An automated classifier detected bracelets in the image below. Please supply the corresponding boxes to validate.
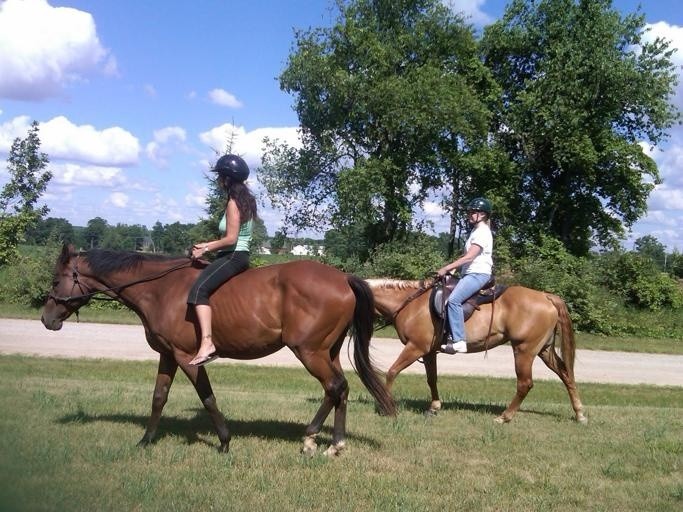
[205,246,209,253]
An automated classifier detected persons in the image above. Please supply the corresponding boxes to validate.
[436,198,494,353]
[187,154,258,369]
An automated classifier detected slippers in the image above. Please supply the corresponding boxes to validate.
[188,354,217,368]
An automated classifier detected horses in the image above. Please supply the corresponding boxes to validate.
[349,278,587,425]
[41,237,401,460]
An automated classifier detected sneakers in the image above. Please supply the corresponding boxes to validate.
[440,340,467,352]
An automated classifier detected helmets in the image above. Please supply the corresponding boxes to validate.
[210,154,249,180]
[467,198,491,212]
